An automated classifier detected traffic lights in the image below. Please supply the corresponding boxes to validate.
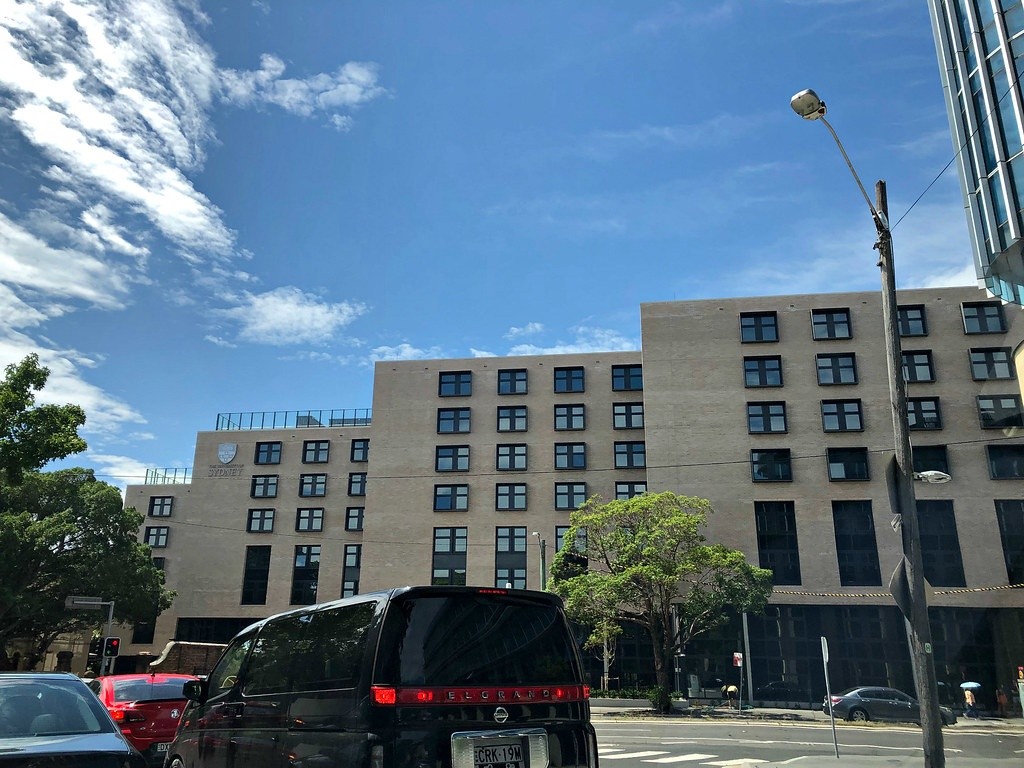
[103,637,120,658]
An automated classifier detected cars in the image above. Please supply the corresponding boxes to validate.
[0,671,151,768]
[87,671,207,761]
[823,684,959,727]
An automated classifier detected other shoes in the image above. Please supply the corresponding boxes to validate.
[963,713,968,719]
[975,718,980,721]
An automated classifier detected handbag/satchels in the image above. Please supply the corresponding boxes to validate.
[999,695,1005,704]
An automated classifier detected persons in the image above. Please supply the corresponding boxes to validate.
[963,688,978,720]
[720,685,728,699]
[726,685,738,709]
[1010,679,1021,718]
[996,683,1007,717]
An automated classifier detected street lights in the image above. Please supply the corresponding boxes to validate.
[790,87,948,768]
[533,531,546,593]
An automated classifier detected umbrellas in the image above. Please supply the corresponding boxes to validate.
[959,682,981,688]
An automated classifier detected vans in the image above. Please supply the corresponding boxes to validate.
[160,582,599,768]
